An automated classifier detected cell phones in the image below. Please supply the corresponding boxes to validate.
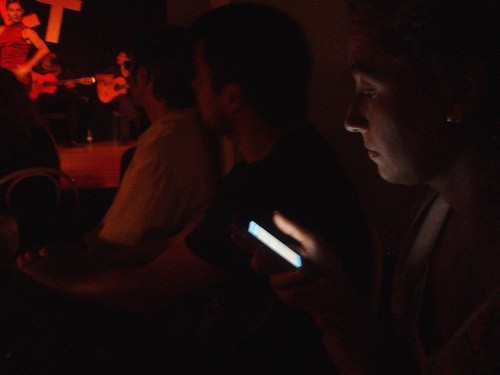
[245,220,312,273]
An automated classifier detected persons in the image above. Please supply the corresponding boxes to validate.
[230,0,500,375]
[0,0,372,375]
